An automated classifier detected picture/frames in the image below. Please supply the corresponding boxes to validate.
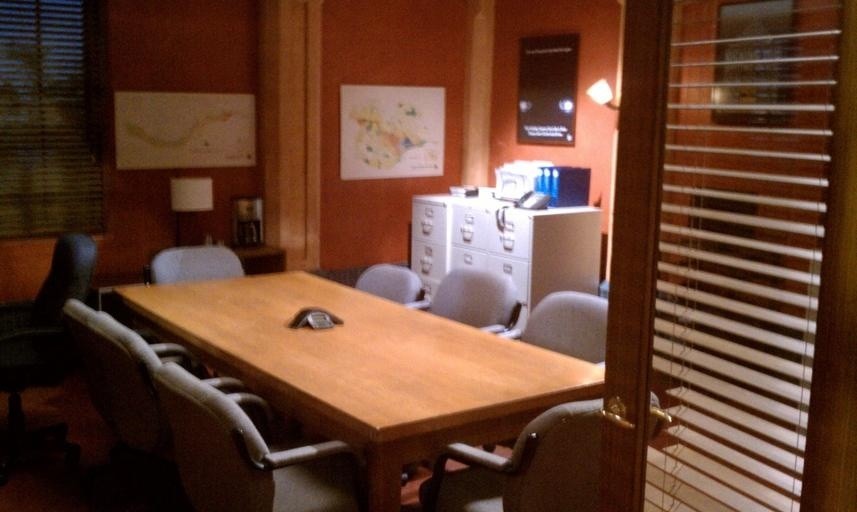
[515,32,579,145]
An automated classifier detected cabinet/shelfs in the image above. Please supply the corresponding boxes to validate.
[409,186,603,320]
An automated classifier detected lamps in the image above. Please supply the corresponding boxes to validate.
[168,176,213,247]
[585,78,617,110]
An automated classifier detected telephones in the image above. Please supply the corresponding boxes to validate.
[514,190,548,209]
[290,306,343,330]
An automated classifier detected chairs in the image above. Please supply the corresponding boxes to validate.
[71,246,607,511]
